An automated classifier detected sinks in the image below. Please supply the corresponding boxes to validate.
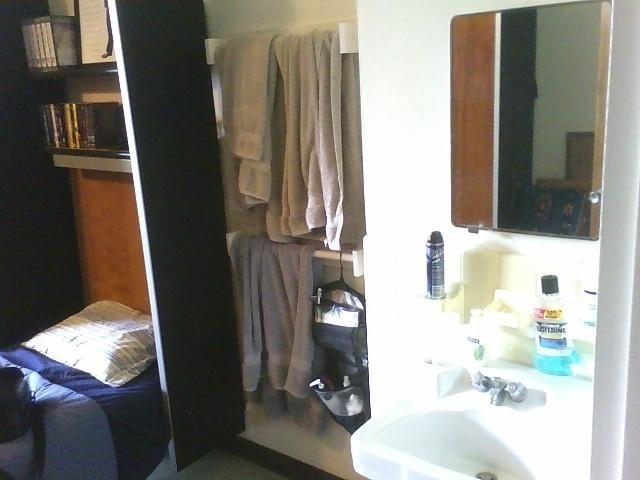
[350,360,594,480]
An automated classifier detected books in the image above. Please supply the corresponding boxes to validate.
[20,14,80,72]
[36,100,119,150]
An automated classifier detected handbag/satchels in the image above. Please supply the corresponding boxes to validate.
[0,367,33,443]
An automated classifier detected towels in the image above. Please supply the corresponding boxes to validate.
[224,233,319,403]
[217,25,279,215]
[272,28,350,252]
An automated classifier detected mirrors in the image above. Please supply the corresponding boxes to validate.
[448,0,615,243]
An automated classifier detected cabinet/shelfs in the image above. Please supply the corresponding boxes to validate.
[26,61,135,174]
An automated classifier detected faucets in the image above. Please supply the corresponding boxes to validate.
[470,372,527,407]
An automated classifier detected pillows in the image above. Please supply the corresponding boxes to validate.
[19,299,163,394]
